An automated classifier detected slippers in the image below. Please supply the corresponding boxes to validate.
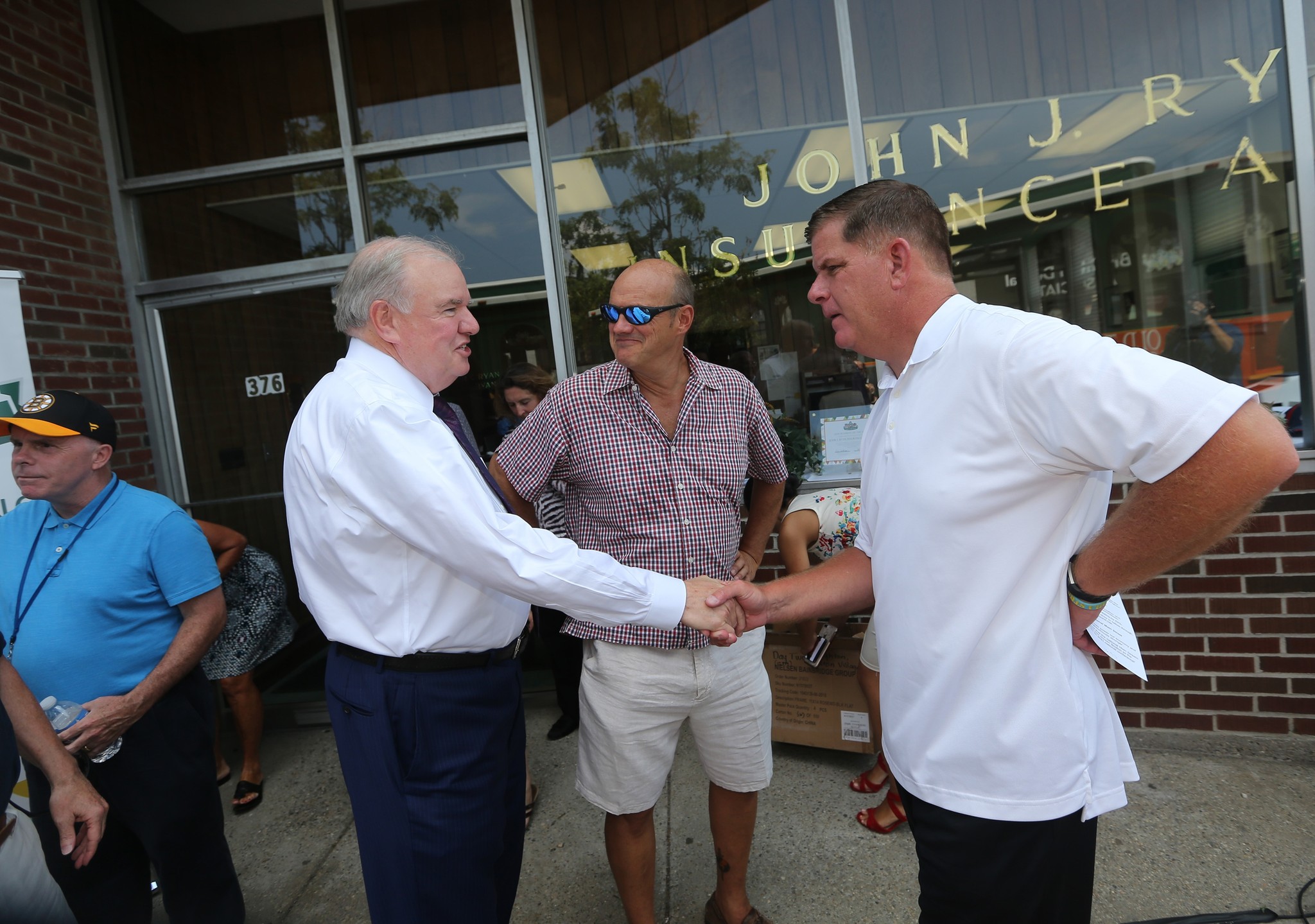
[217,771,231,787]
[231,779,264,812]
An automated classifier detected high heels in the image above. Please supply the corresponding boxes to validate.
[850,751,895,793]
[856,790,908,834]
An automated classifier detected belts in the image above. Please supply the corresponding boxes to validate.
[328,620,532,672]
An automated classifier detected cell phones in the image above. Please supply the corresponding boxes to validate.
[802,623,837,667]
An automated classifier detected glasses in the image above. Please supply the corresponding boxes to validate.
[599,302,682,326]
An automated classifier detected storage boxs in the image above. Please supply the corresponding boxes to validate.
[763,622,876,754]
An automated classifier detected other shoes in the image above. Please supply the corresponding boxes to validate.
[547,709,580,740]
[703,890,774,924]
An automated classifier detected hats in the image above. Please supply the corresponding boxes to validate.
[0,389,119,450]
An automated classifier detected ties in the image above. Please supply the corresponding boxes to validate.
[431,394,516,516]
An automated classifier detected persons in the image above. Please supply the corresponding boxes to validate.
[0,392,247,924]
[744,472,913,832]
[283,232,748,924]
[701,179,1300,924]
[491,256,788,924]
[1045,300,1064,320]
[0,632,110,924]
[497,361,583,743]
[186,517,286,811]
[1163,286,1246,390]
[728,321,870,433]
[522,749,533,830]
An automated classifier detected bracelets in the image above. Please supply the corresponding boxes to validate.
[1060,552,1120,612]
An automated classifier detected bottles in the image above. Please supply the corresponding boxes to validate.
[39,696,122,763]
